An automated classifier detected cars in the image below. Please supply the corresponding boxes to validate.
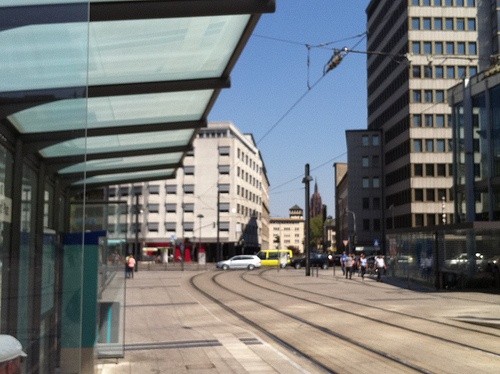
[216,254,261,270]
[291,253,342,271]
[364,251,496,281]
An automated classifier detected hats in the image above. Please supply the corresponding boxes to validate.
[0,334,28,363]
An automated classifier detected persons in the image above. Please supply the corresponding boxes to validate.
[0,333,28,374]
[421,256,433,281]
[125,255,135,279]
[374,254,386,282]
[110,252,121,265]
[340,251,366,280]
[390,254,410,277]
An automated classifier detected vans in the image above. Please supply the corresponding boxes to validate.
[257,250,293,266]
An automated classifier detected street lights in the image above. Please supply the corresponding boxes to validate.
[134,191,142,271]
[343,208,357,244]
[197,214,205,247]
